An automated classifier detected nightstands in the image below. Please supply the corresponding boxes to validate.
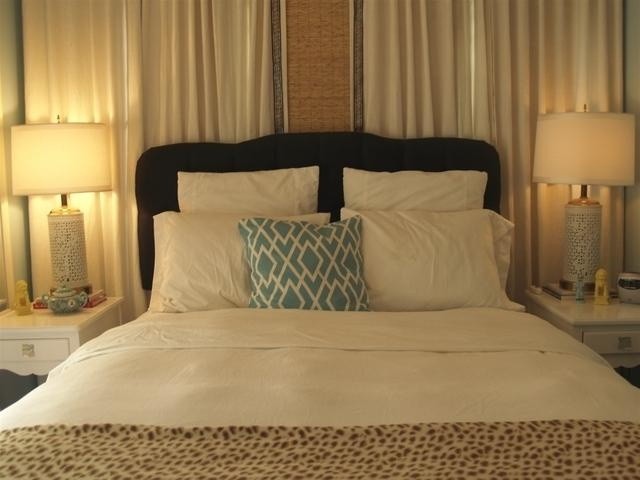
[525,286,640,368]
[0,296,125,376]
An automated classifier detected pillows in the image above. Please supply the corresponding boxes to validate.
[343,166,488,211]
[340,208,526,312]
[147,210,330,313]
[177,166,319,214]
[239,215,371,312]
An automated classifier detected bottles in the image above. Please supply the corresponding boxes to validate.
[576,273,585,303]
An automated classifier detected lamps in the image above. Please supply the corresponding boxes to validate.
[532,104,636,301]
[11,114,112,307]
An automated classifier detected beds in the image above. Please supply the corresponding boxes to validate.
[0,131,640,479]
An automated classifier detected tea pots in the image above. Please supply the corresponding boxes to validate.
[42,284,88,314]
